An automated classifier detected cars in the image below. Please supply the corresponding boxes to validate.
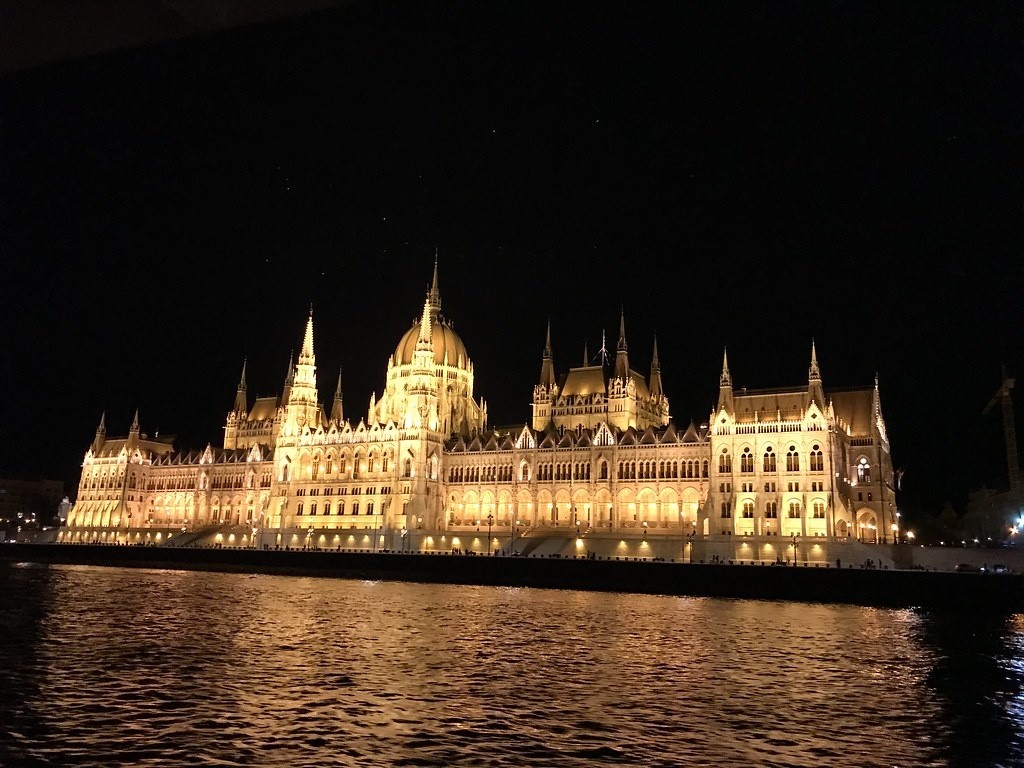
[954,562,976,572]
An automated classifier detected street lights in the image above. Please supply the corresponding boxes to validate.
[307,517,314,552]
[372,511,377,553]
[686,530,696,564]
[487,509,494,557]
[790,534,799,567]
[251,518,259,550]
